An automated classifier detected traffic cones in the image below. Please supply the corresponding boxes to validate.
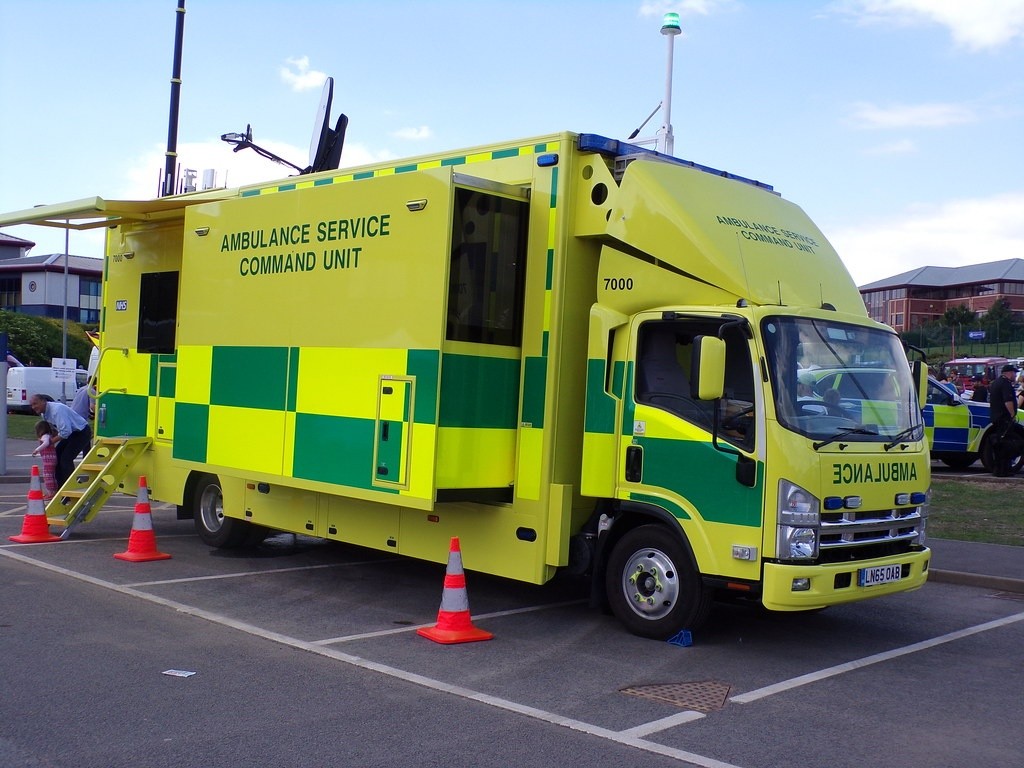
[8,465,76,544]
[416,537,494,644]
[112,476,176,562]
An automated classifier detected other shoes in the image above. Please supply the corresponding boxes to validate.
[993,470,1014,477]
[43,493,55,501]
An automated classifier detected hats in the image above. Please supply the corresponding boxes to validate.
[968,373,982,382]
[1001,365,1020,372]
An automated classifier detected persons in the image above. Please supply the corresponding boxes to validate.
[796,375,855,418]
[31,375,97,502]
[925,364,1023,478]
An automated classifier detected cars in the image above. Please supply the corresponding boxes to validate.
[944,355,1024,402]
[796,365,1024,477]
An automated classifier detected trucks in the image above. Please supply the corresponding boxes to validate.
[5,366,88,416]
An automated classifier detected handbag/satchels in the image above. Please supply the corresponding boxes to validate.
[955,379,966,394]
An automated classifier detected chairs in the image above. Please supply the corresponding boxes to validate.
[641,332,712,420]
[726,326,756,402]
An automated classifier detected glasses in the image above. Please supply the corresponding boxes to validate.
[950,372,953,374]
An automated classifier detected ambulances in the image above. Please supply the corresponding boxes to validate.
[0,11,933,640]
